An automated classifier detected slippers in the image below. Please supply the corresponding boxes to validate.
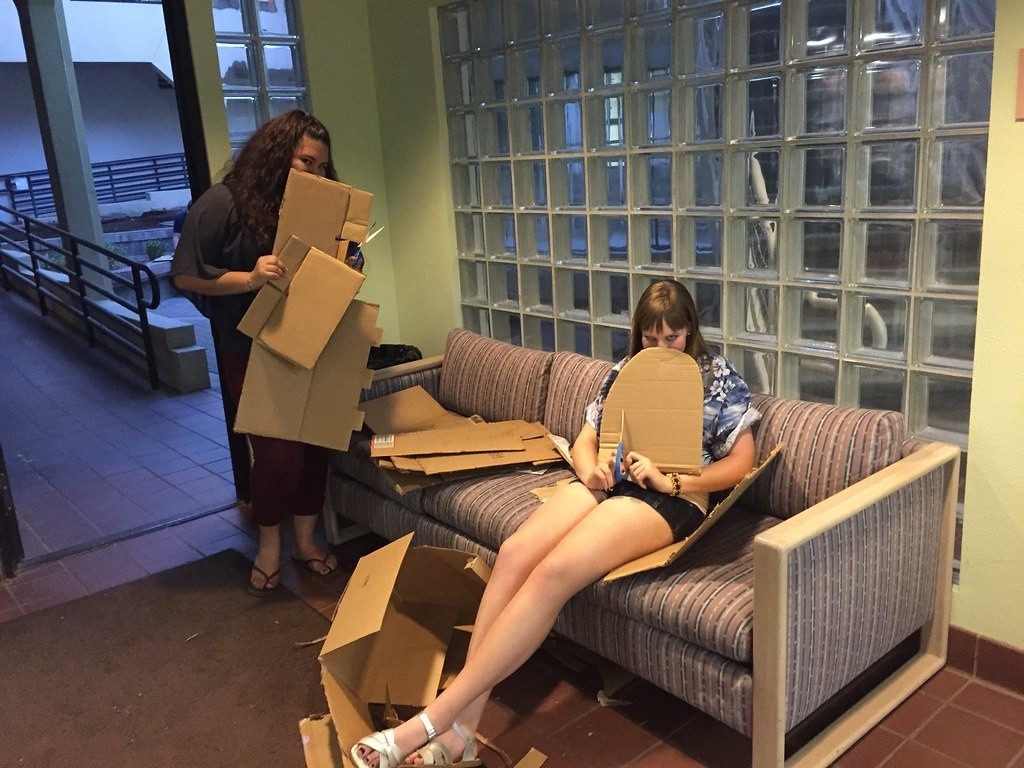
[290,550,340,575]
[247,560,281,595]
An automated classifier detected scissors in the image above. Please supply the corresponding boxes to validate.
[614,409,626,482]
[346,221,386,268]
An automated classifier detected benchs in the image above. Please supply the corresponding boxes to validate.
[325,327,961,768]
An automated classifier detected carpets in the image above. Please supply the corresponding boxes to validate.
[0,548,336,768]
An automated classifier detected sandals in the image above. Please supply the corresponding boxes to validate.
[351,712,437,768]
[398,722,482,768]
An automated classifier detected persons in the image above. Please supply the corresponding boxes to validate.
[167,109,364,598]
[349,279,763,768]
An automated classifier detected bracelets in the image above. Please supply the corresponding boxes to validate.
[666,472,683,496]
[247,271,253,290]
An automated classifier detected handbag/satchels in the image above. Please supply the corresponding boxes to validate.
[366,344,422,370]
